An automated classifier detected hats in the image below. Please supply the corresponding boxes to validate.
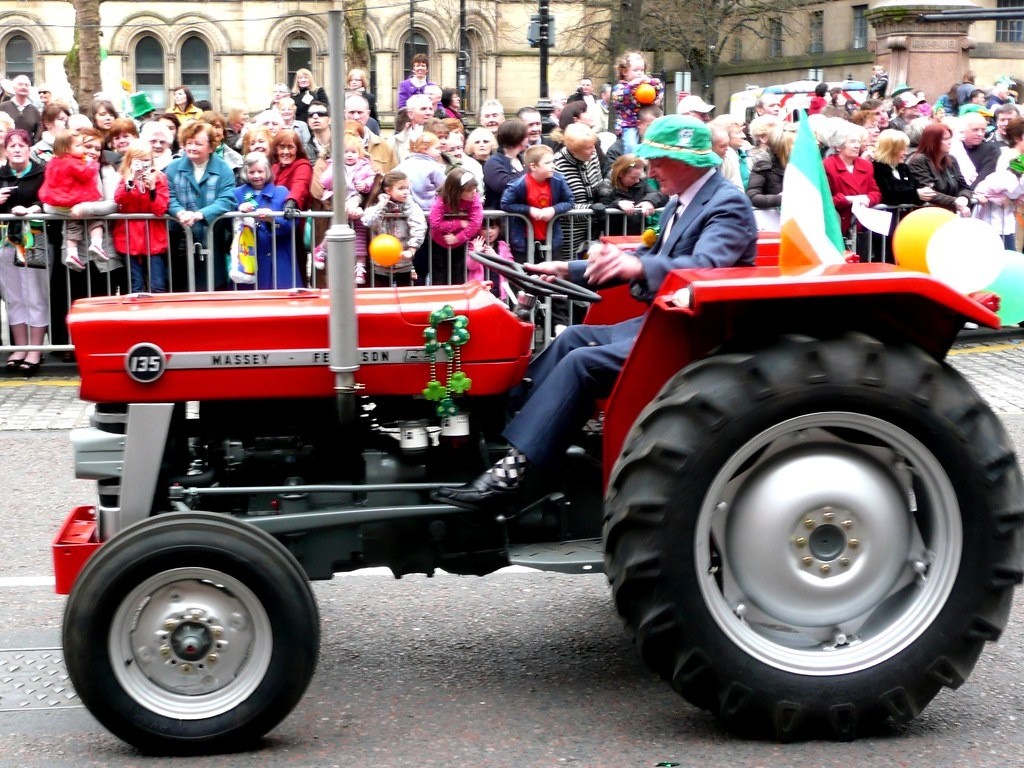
[128,91,158,118]
[634,114,724,166]
[891,83,914,98]
[676,95,718,116]
[893,91,927,111]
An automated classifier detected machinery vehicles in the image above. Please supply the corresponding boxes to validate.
[49,10,1024,762]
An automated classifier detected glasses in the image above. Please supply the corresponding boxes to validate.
[37,90,52,95]
[306,110,330,119]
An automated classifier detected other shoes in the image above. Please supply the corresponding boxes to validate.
[64,255,85,270]
[314,245,327,270]
[88,244,109,264]
[964,321,979,330]
[355,265,366,284]
[5,352,45,375]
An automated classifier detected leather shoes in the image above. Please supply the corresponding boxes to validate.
[432,472,530,515]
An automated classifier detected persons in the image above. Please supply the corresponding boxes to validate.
[431,115,755,512]
[0,0,1024,376]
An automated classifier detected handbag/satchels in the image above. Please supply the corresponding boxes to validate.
[228,217,259,284]
[14,231,50,269]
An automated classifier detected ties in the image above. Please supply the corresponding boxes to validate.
[671,202,683,230]
